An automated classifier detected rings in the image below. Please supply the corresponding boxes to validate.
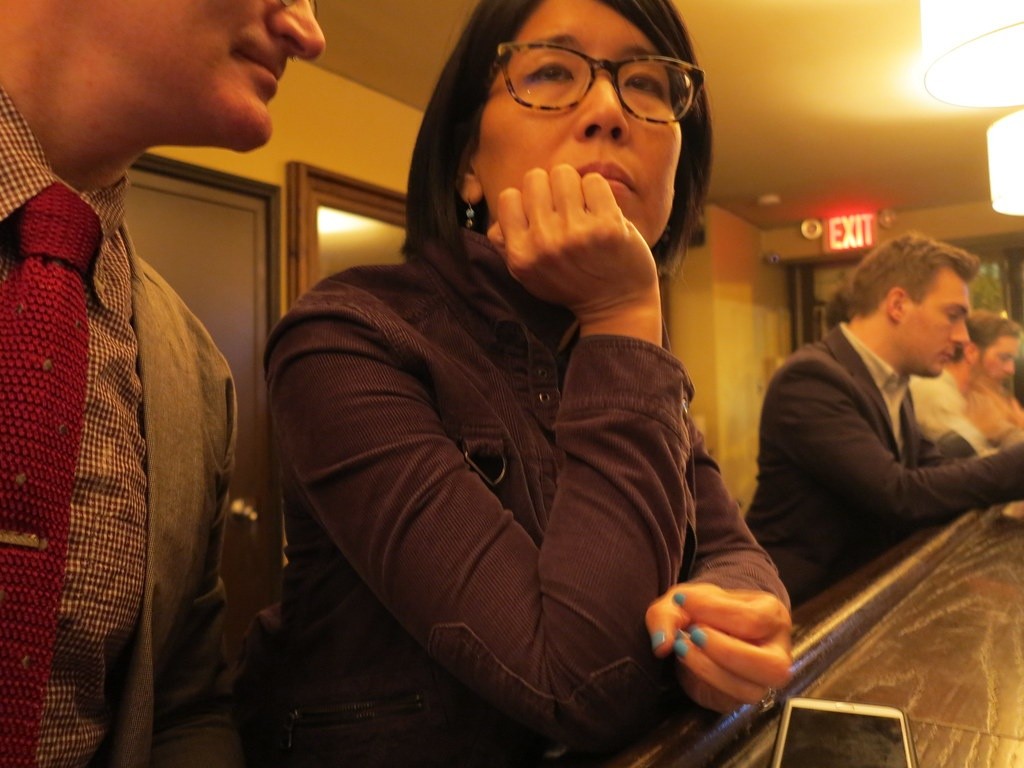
[756,687,774,713]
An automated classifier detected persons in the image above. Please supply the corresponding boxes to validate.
[0,1,327,768]
[743,228,1024,606]
[260,0,799,768]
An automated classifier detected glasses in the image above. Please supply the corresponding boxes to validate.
[485,42,706,124]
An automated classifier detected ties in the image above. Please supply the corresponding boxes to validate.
[0,183,103,768]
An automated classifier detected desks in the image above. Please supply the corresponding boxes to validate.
[609,495,1024,768]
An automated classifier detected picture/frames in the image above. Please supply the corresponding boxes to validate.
[285,160,409,314]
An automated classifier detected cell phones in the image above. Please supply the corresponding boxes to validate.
[770,696,920,768]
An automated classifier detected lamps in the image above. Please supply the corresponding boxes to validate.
[917,0,1024,217]
[798,209,897,252]
